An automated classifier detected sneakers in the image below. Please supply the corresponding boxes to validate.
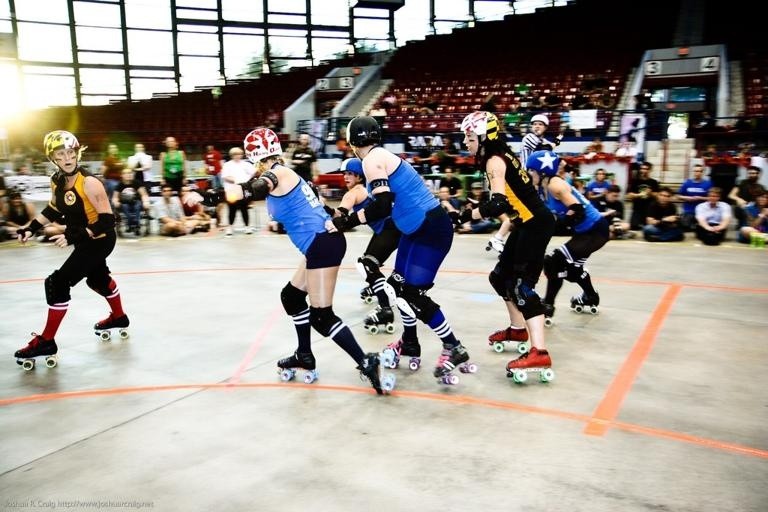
[226,227,233,235]
[243,225,252,234]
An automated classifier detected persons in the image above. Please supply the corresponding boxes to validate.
[306,158,396,324]
[269,82,767,245]
[14,129,131,358]
[525,148,610,318]
[325,115,477,377]
[227,125,384,394]
[1,137,289,244]
[441,110,558,372]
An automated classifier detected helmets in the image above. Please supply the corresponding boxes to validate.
[339,158,365,178]
[526,150,560,176]
[530,114,550,127]
[346,116,382,147]
[43,130,81,162]
[460,110,501,142]
[244,128,283,164]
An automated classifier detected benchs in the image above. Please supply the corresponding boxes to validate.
[366,65,632,134]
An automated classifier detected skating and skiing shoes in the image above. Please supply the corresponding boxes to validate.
[382,331,421,371]
[434,340,477,385]
[540,299,554,327]
[363,306,395,335]
[570,293,599,315]
[360,286,377,304]
[94,312,129,341]
[277,349,319,384]
[15,332,57,371]
[506,347,554,383]
[485,235,506,260]
[356,349,395,395]
[488,326,529,354]
